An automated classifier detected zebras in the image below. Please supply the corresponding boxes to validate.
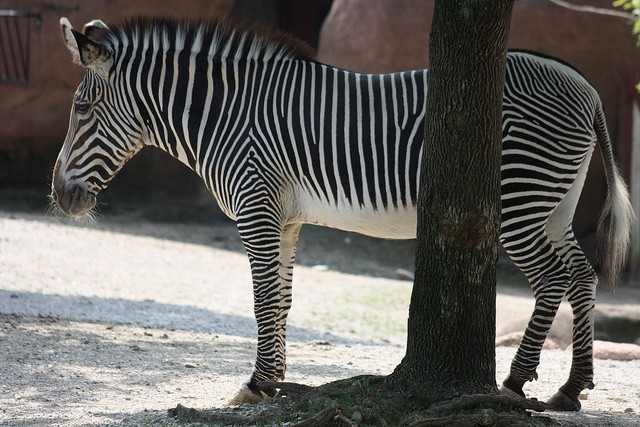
[50,14,639,411]
